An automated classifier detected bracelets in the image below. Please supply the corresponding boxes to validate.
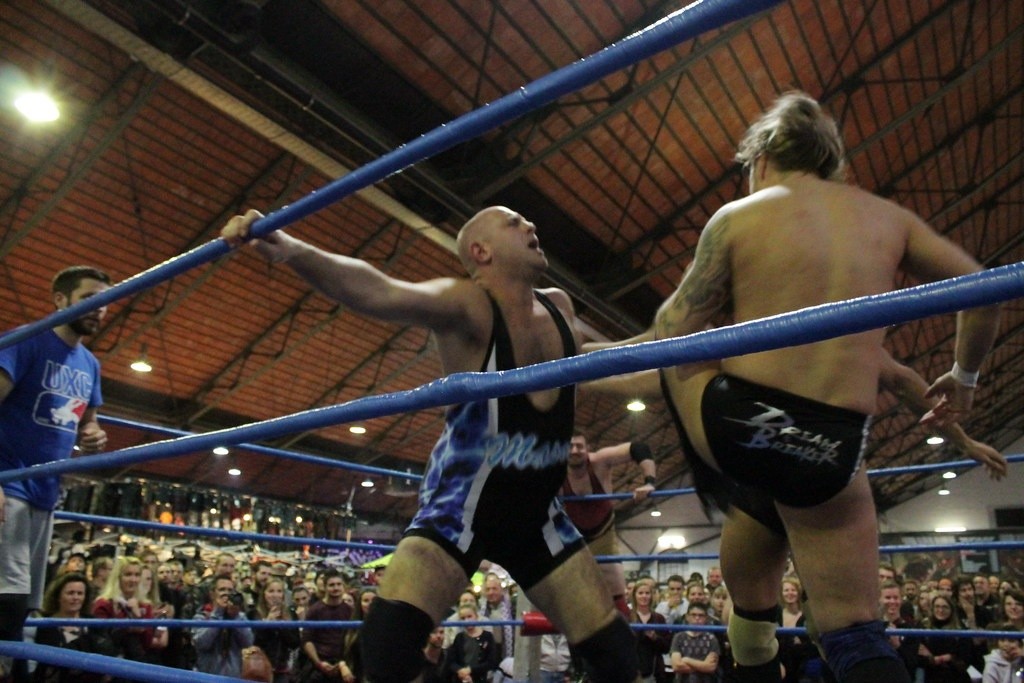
[644,474,655,485]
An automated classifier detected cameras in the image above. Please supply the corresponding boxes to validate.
[221,592,244,606]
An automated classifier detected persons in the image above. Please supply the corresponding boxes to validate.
[0,264,111,682]
[221,205,649,682]
[550,431,656,622]
[577,92,1023,682]
[33,535,1024,683]
